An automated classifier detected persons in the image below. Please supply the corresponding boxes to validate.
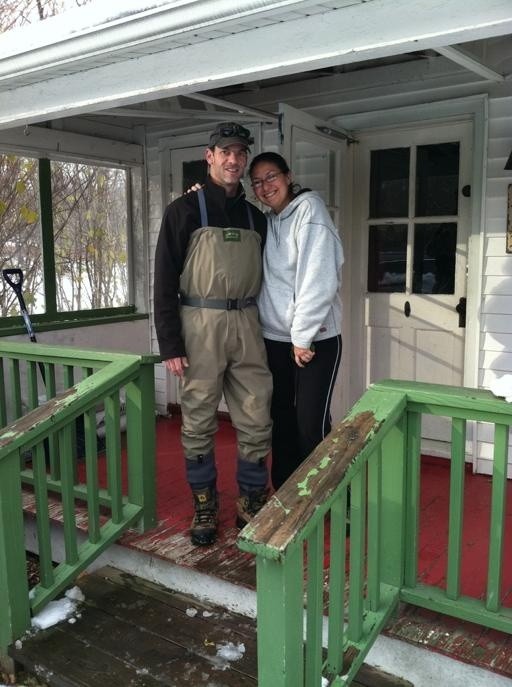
[154,118,275,547]
[185,151,351,538]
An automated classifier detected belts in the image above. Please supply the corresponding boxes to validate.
[181,295,254,310]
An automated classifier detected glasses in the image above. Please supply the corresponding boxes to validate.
[219,127,250,137]
[251,173,281,187]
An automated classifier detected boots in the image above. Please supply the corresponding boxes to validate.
[191,486,218,545]
[236,488,270,530]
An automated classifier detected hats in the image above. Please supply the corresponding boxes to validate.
[209,122,251,153]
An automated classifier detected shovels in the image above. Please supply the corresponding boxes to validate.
[2,268,105,459]
